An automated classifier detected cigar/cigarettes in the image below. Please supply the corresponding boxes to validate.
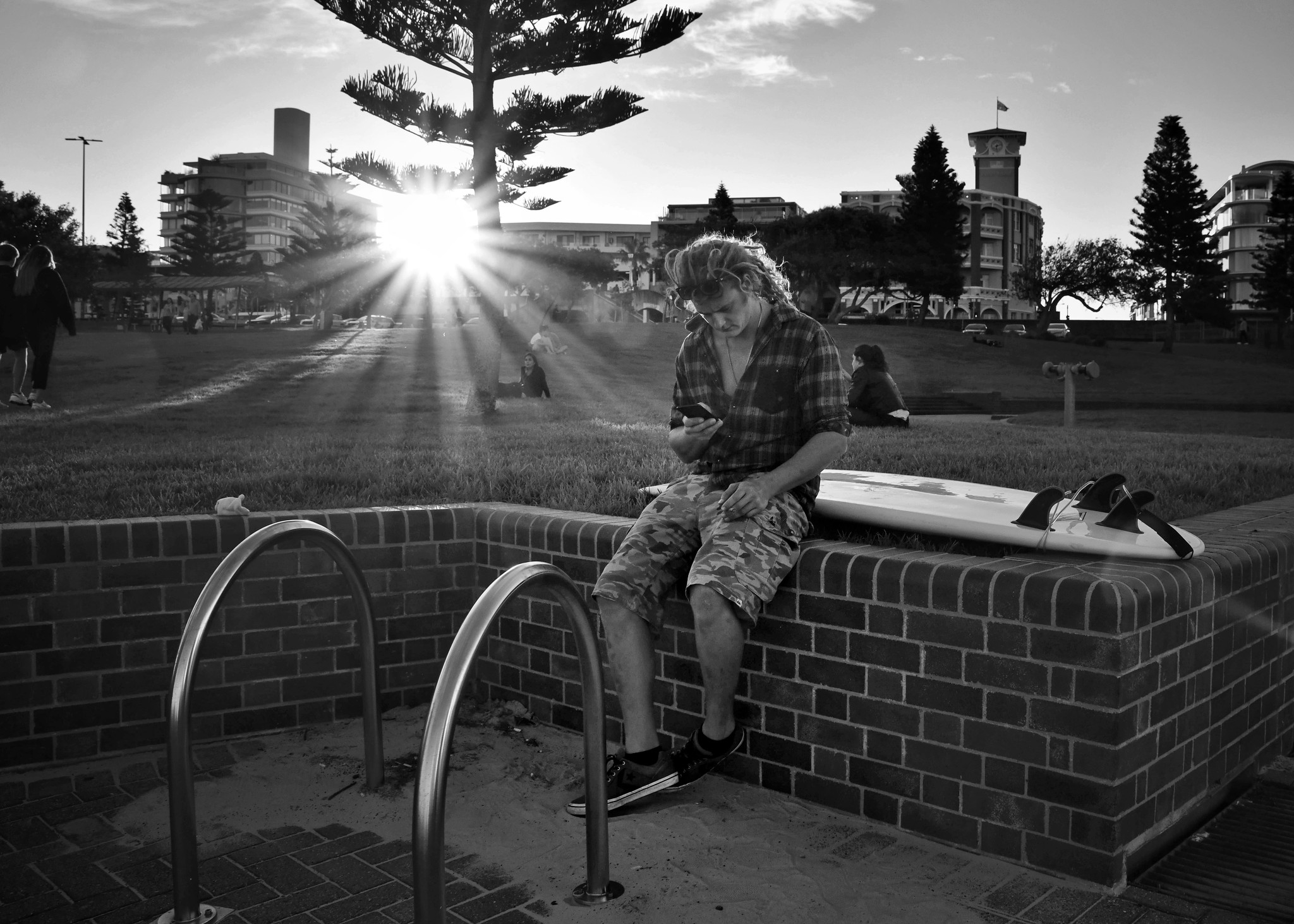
[728,508,736,513]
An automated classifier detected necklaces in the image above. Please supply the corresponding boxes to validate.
[725,303,762,387]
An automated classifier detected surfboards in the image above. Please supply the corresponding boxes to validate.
[637,468,1205,560]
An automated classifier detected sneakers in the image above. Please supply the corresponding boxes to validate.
[567,751,680,816]
[31,401,51,408]
[654,721,744,794]
[9,392,29,406]
[27,392,36,406]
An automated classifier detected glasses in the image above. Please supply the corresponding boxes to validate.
[676,276,738,301]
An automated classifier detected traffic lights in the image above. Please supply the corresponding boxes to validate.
[669,292,674,301]
[968,302,972,309]
[974,313,978,318]
[953,296,958,307]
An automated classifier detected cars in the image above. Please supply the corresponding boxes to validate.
[1048,323,1070,338]
[244,314,403,329]
[195,313,224,326]
[962,323,986,336]
[1003,325,1026,336]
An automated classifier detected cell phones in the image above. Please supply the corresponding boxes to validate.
[676,404,717,422]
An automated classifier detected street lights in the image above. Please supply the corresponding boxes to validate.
[66,137,103,255]
[628,253,633,290]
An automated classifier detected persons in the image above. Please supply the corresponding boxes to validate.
[498,352,551,399]
[567,231,854,816]
[107,293,214,335]
[528,325,568,355]
[313,304,333,331]
[842,344,911,428]
[1236,316,1250,345]
[0,244,77,410]
